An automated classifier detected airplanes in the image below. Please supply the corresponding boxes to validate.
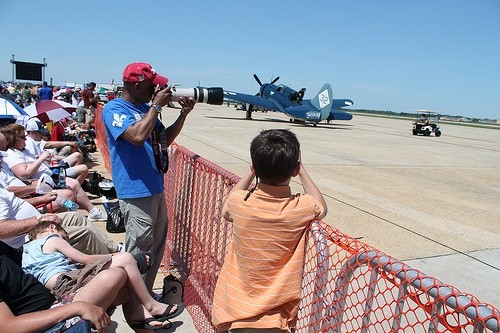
[222,72,354,126]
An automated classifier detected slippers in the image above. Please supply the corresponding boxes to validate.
[134,252,155,272]
[131,318,172,330]
[156,303,185,321]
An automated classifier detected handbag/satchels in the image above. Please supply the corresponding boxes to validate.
[88,172,103,194]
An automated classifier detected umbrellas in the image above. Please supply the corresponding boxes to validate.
[15,100,78,126]
[0,98,28,118]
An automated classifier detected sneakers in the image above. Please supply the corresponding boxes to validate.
[87,211,108,222]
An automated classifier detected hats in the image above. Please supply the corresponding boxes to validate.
[89,82,96,87]
[123,62,169,87]
[27,119,49,136]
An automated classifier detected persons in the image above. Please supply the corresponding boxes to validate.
[102,62,197,292]
[419,114,426,119]
[211,128,328,333]
[0,82,185,333]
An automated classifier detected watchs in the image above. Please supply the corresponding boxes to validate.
[153,105,161,112]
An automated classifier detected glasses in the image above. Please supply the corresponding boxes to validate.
[17,136,26,139]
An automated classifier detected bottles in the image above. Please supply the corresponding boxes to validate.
[51,155,59,174]
[51,148,58,157]
[53,195,79,211]
[58,165,66,189]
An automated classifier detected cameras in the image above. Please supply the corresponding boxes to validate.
[156,84,223,105]
